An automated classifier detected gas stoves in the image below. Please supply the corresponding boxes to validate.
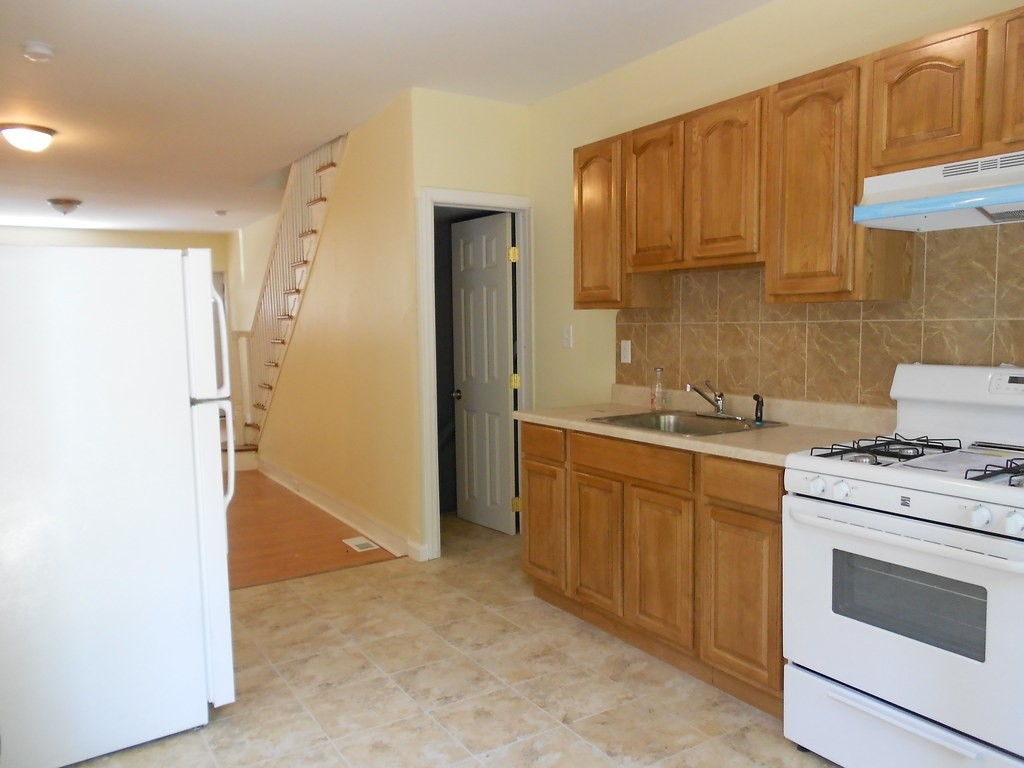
[783,361,1024,541]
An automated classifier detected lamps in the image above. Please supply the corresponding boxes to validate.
[1,123,55,154]
[45,198,81,215]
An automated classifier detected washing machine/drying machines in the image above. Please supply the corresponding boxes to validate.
[782,362,1024,768]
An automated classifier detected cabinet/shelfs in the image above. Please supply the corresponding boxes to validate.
[625,94,761,267]
[624,481,696,650]
[577,139,623,302]
[768,65,859,295]
[1001,16,1024,144]
[871,27,987,169]
[519,455,624,618]
[698,503,782,693]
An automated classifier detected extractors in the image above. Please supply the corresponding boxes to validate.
[851,150,1024,234]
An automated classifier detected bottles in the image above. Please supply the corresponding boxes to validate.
[650,368,670,411]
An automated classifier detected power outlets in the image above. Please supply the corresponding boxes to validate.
[620,339,632,363]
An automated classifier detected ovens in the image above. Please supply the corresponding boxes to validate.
[782,495,1024,768]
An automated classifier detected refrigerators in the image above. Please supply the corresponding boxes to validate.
[0,246,245,768]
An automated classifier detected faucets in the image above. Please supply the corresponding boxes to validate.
[686,379,725,413]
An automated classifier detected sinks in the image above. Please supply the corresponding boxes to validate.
[585,409,786,438]
